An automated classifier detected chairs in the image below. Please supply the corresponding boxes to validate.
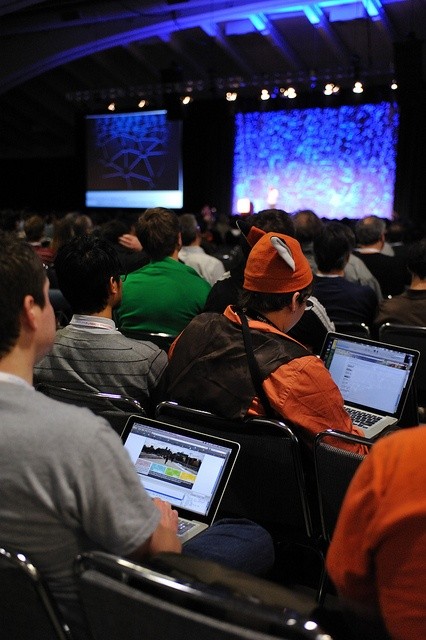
[312,428,376,546]
[73,542,335,640]
[152,399,328,607]
[331,320,371,338]
[377,322,425,406]
[117,327,178,351]
[32,382,145,435]
[0,544,73,640]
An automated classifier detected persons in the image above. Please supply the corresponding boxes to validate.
[352,214,405,298]
[204,208,338,356]
[24,215,56,265]
[177,214,225,287]
[0,208,24,238]
[102,222,143,258]
[112,207,212,338]
[288,210,383,301]
[167,234,371,457]
[45,213,101,237]
[373,239,426,334]
[310,221,378,333]
[33,238,167,418]
[326,419,426,639]
[387,224,408,269]
[0,237,277,640]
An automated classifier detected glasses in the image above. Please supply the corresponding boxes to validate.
[118,269,129,282]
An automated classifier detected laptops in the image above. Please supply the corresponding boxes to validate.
[118,415,242,543]
[318,330,421,447]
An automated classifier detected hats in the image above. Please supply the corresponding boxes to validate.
[236,218,314,294]
